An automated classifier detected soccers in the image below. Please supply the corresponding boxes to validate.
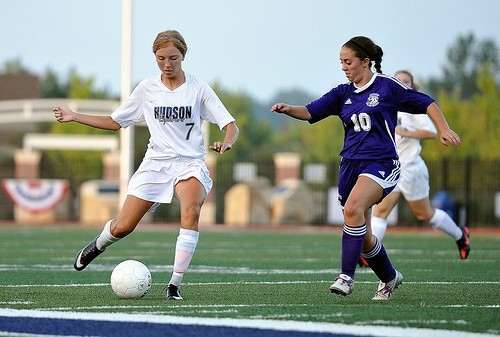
[111,260,152,299]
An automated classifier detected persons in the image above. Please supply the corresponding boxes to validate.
[358,71,470,266]
[269,36,461,301]
[52,30,240,300]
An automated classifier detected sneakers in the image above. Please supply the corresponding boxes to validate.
[329,274,355,296]
[358,254,370,269]
[455,225,471,259]
[370,270,404,301]
[73,235,107,271]
[162,283,183,301]
